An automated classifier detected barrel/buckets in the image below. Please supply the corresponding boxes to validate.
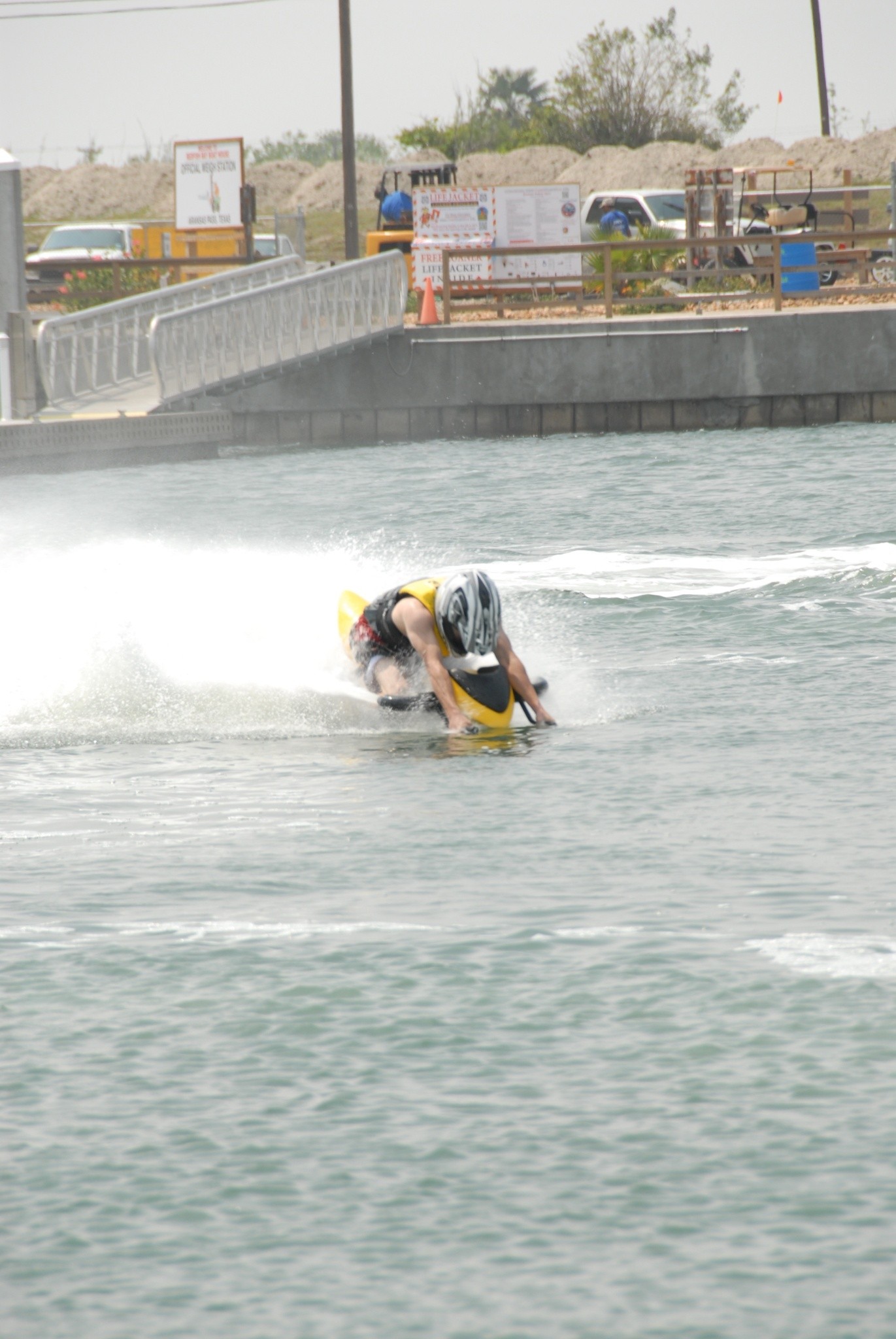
[780,241,819,291]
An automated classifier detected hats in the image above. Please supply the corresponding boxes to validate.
[599,198,615,209]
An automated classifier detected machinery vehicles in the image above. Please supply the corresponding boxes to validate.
[364,159,460,291]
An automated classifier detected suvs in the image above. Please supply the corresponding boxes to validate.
[24,221,144,303]
[580,187,775,284]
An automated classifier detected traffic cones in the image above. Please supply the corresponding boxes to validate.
[414,277,443,326]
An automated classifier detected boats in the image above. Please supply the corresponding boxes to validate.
[338,588,549,730]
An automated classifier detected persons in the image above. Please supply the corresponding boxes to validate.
[349,570,554,732]
[597,199,631,237]
[375,187,413,222]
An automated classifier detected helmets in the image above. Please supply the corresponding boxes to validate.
[435,570,502,659]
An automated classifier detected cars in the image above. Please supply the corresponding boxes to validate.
[254,233,296,256]
